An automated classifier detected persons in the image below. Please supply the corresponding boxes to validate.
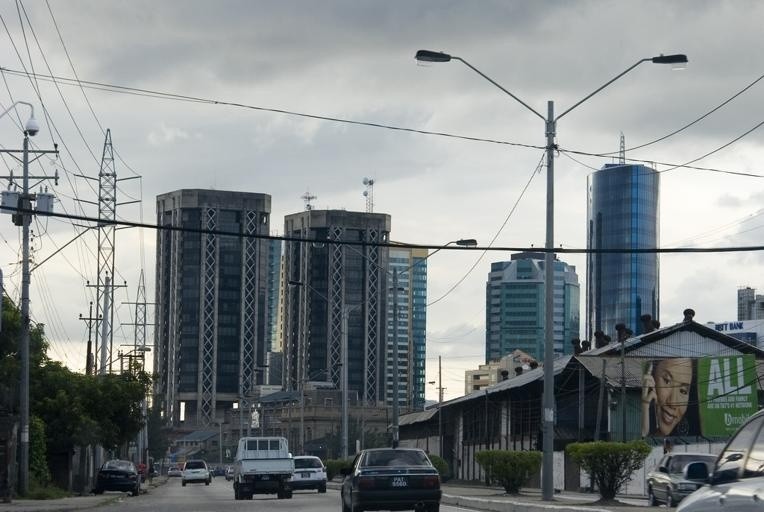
[642,358,698,436]
[137,461,156,484]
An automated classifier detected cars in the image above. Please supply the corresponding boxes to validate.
[671,407,764,512]
[93,456,141,498]
[644,450,722,508]
[146,457,235,488]
[336,445,443,511]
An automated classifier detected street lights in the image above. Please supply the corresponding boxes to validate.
[233,395,252,435]
[19,220,117,495]
[313,233,476,445]
[412,46,692,507]
[255,362,344,453]
[90,345,151,375]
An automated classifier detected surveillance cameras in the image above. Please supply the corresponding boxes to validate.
[26,118,40,136]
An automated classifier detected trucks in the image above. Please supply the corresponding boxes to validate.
[233,436,294,501]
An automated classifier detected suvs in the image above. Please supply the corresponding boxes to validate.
[285,455,329,494]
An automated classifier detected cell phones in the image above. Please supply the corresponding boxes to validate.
[646,360,659,432]
